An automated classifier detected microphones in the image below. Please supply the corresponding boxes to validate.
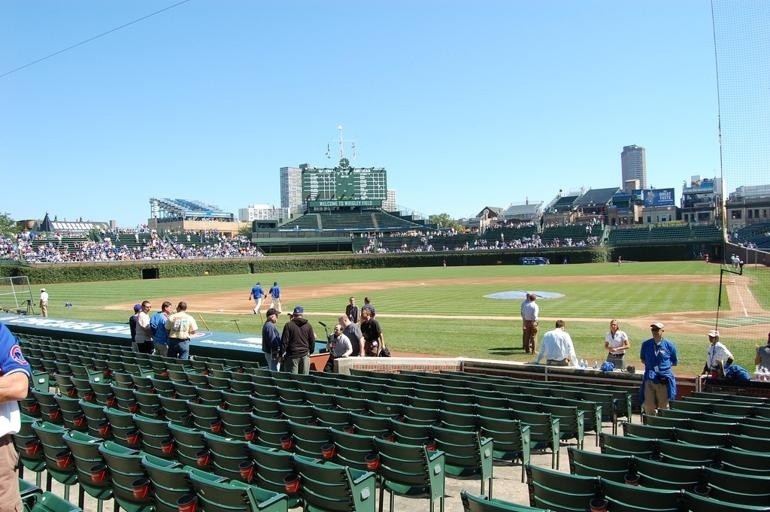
[319,321,326,328]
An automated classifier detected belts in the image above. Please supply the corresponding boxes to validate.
[609,352,624,356]
[0,433,14,447]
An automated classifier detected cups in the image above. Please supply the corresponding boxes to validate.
[754,371,770,382]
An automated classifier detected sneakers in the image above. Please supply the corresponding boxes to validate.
[40,288,46,292]
[253,309,261,314]
[524,344,539,354]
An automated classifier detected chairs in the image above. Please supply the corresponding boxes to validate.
[142,352,376,511]
[10,332,142,512]
[377,362,770,512]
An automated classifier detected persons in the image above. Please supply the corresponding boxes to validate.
[249,281,267,314]
[39,288,49,317]
[0,230,264,264]
[730,229,758,275]
[545,255,550,265]
[265,282,282,312]
[261,296,386,374]
[562,255,568,265]
[0,319,32,512]
[617,255,622,266]
[443,258,448,269]
[129,300,198,360]
[704,252,710,263]
[356,217,600,254]
[521,292,770,416]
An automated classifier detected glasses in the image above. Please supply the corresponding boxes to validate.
[651,328,663,331]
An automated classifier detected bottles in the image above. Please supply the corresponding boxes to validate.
[593,360,599,371]
[580,359,584,367]
[584,359,588,368]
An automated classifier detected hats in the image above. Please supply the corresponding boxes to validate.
[267,308,281,316]
[650,322,664,328]
[707,330,720,337]
[294,305,304,314]
[134,304,142,313]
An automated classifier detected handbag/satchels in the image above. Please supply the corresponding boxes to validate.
[378,346,391,357]
